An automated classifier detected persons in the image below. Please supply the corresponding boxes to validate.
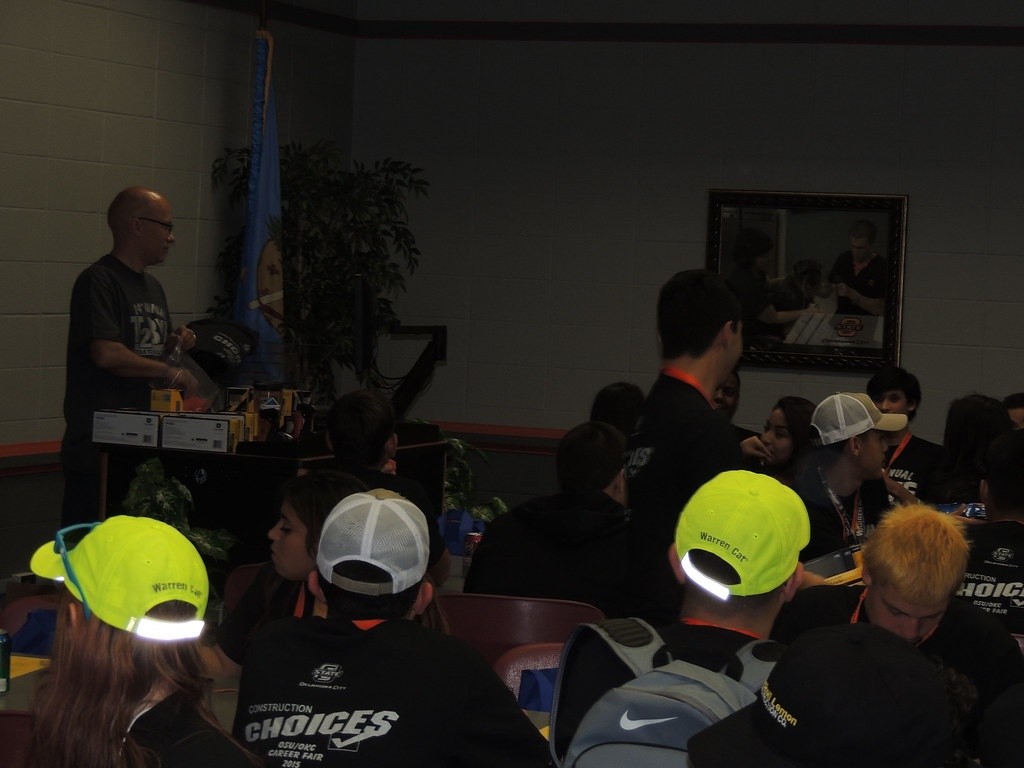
[201,389,554,768]
[29,515,262,768]
[713,366,1024,633]
[688,622,981,768]
[722,218,887,350]
[461,383,642,619]
[769,503,1024,767]
[663,469,812,694]
[626,270,824,625]
[58,187,198,538]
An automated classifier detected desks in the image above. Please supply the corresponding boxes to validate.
[97,440,450,557]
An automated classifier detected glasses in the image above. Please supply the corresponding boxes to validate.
[54,521,104,620]
[137,217,174,233]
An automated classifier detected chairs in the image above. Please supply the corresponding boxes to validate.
[0,560,605,768]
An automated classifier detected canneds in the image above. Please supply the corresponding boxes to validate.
[224,386,250,412]
[0,630,11,696]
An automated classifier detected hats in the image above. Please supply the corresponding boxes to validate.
[811,392,907,445]
[676,470,810,600]
[317,489,430,593]
[686,621,942,768]
[660,268,758,341]
[30,515,208,640]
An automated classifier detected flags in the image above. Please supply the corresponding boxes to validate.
[235,32,285,386]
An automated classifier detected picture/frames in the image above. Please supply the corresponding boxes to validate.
[707,186,908,373]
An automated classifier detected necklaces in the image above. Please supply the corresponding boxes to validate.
[852,585,938,647]
[680,616,762,640]
